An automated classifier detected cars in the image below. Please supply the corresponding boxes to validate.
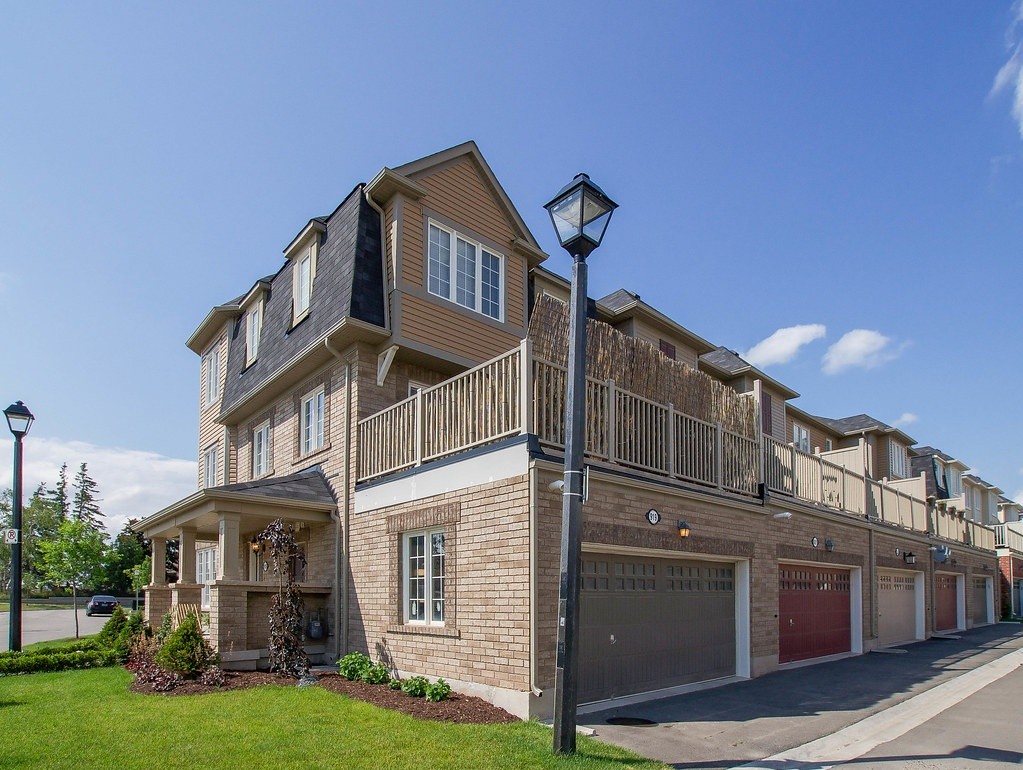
[87,595,118,617]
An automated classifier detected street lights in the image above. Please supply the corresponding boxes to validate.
[5,400,36,656]
[133,569,139,613]
[542,173,622,758]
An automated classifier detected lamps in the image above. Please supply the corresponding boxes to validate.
[825,538,834,552]
[902,551,917,564]
[678,519,691,539]
[251,536,261,555]
